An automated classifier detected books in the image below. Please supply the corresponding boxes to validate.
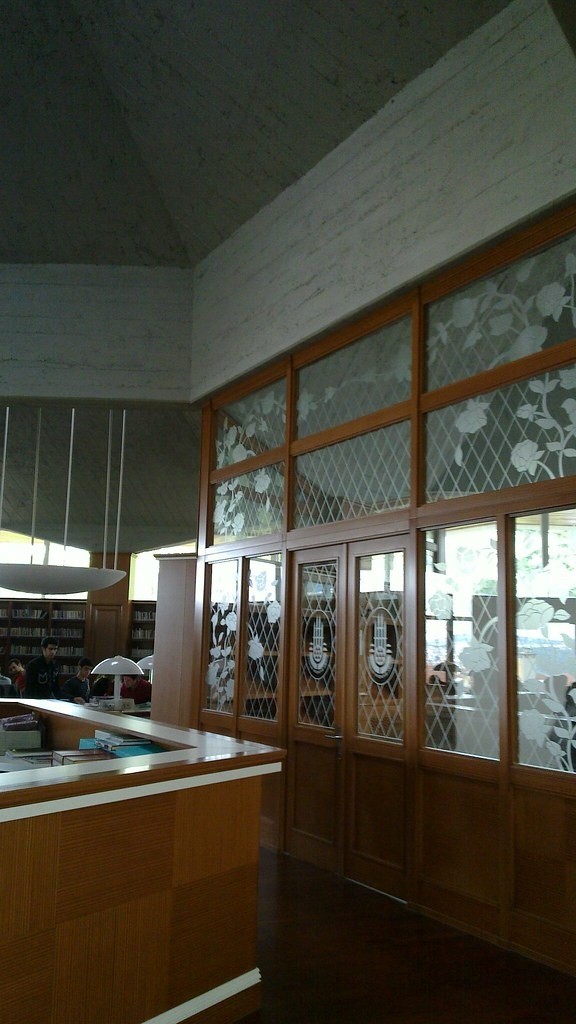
[0,609,83,675]
[132,610,156,676]
[94,728,152,745]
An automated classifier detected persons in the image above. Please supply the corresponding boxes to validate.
[7,658,26,691]
[91,675,152,705]
[22,637,61,699]
[62,657,93,705]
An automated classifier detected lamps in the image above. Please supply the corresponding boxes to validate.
[137,654,154,684]
[0,403,128,597]
[91,655,143,711]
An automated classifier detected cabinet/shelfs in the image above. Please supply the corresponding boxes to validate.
[0,598,87,676]
[130,601,156,663]
[205,592,406,744]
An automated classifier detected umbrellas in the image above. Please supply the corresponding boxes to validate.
[136,654,154,670]
[91,656,144,709]
